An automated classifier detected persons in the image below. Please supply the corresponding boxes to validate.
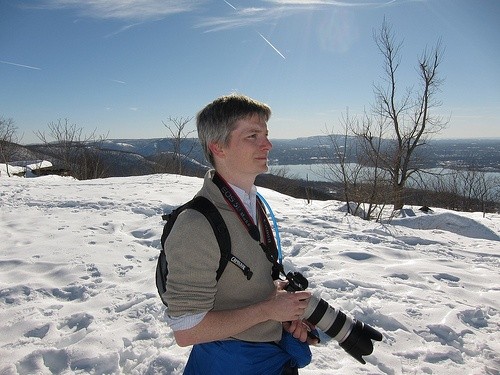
[159,94,322,375]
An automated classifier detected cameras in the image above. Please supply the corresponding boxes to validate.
[282,270,382,365]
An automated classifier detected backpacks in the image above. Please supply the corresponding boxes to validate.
[152,196,233,307]
